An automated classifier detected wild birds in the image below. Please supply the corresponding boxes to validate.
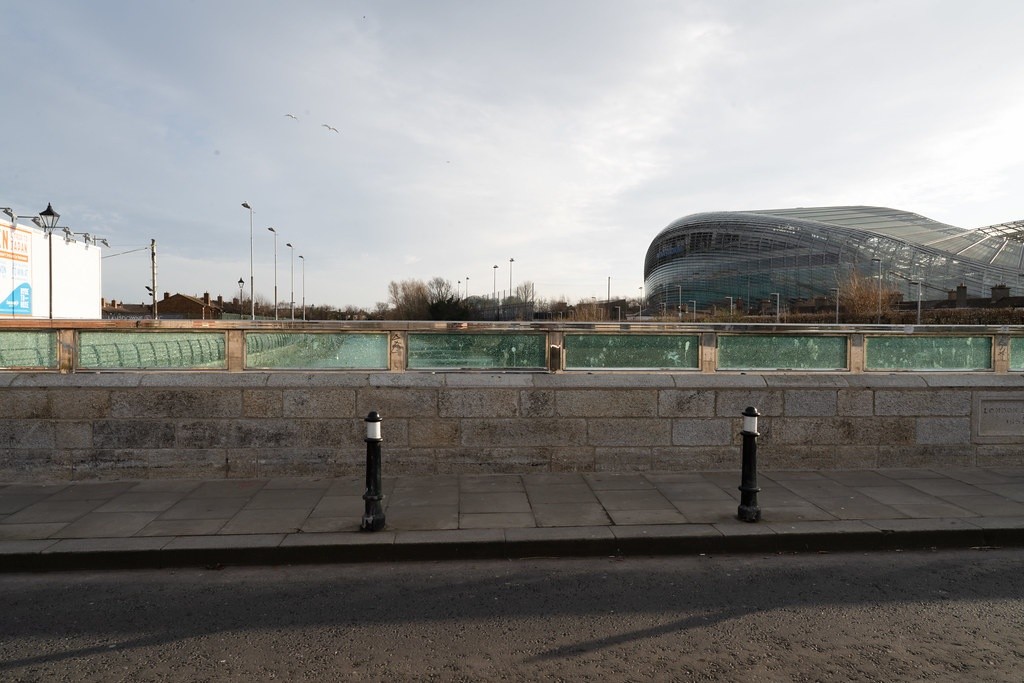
[285,113,298,121]
[321,124,340,135]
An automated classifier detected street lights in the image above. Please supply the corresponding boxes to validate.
[829,287,840,325]
[297,254,305,320]
[39,202,60,319]
[769,292,780,322]
[509,257,514,296]
[492,265,498,297]
[240,201,254,319]
[871,257,882,324]
[673,284,682,323]
[266,227,279,321]
[456,276,470,300]
[286,242,295,320]
[237,276,246,319]
[724,296,733,316]
[907,280,922,324]
